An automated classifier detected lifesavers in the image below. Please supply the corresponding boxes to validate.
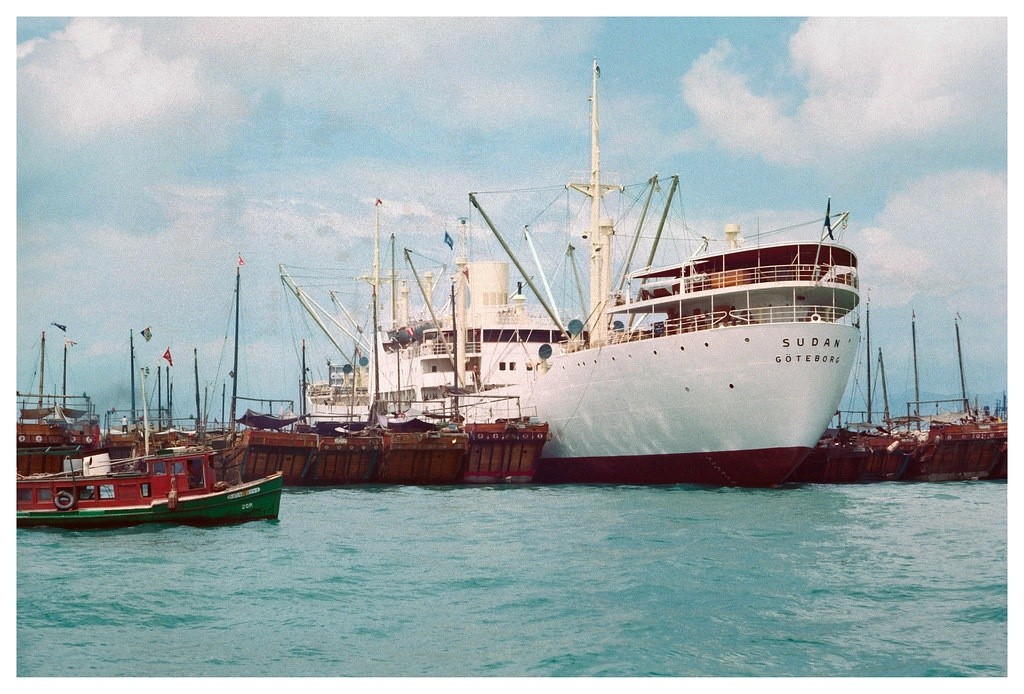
[54,490,75,510]
[69,436,76,443]
[18,435,26,442]
[85,436,93,443]
[36,436,43,442]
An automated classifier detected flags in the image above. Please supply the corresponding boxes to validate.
[140,327,153,343]
[162,347,173,367]
[444,231,454,251]
[237,254,244,267]
[51,322,77,347]
[824,196,835,241]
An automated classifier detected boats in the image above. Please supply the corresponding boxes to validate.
[301,59,865,490]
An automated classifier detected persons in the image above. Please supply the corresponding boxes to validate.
[120,416,129,433]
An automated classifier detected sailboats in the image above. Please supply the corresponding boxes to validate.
[792,304,1007,484]
[16,199,555,529]
